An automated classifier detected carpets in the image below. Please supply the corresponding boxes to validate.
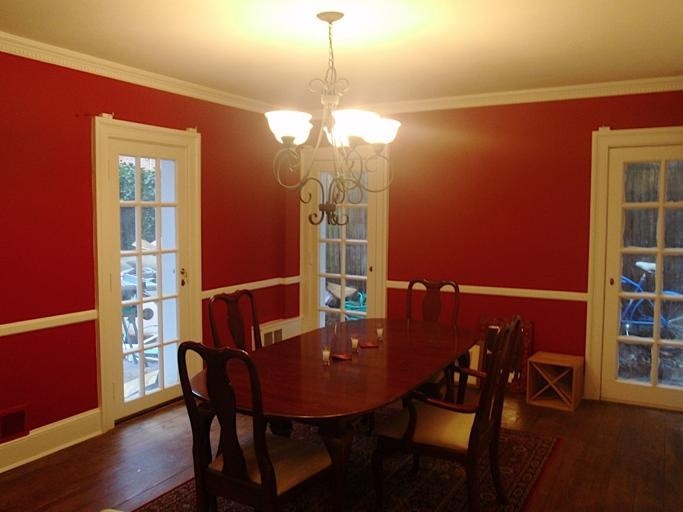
[130,424,562,512]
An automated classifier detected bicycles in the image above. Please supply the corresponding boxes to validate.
[324,281,366,327]
[619,260,682,358]
[121,283,158,393]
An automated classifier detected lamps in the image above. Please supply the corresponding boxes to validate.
[262,11,399,225]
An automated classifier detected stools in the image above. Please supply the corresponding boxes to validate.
[526,351,585,413]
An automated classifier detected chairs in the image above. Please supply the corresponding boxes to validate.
[209,289,261,355]
[404,279,468,404]
[177,341,331,511]
[372,315,518,509]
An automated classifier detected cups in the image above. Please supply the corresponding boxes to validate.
[320,342,332,367]
[373,324,385,342]
[347,334,359,353]
